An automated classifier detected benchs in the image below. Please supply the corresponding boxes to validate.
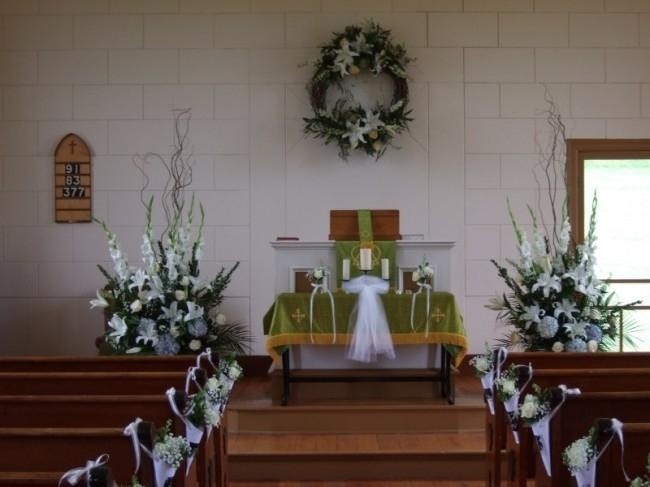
[484,352,650,485]
[1,355,229,487]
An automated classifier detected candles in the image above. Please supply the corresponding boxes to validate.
[382,258,389,279]
[343,260,350,280]
[360,248,372,270]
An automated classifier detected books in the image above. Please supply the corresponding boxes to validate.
[400,233,425,241]
[277,236,299,241]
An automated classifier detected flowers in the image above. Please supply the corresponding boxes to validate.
[301,17,428,166]
[412,252,434,289]
[219,357,244,383]
[305,259,332,290]
[467,354,493,374]
[482,81,647,352]
[90,105,260,355]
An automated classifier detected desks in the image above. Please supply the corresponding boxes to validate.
[274,291,455,405]
